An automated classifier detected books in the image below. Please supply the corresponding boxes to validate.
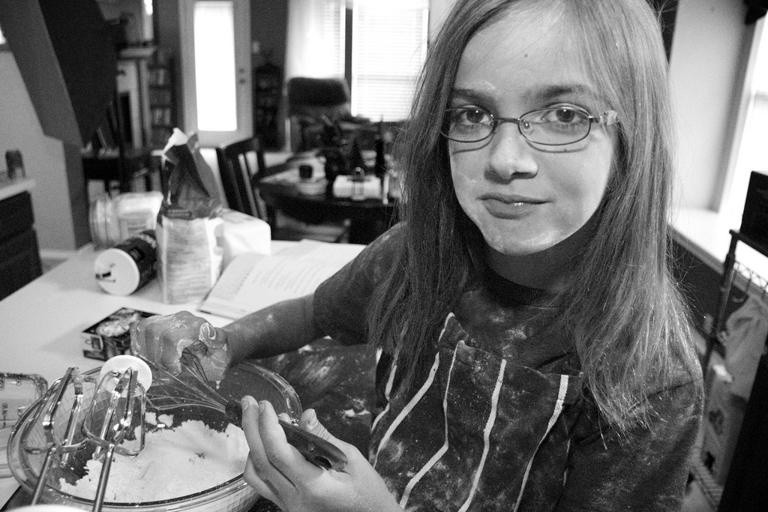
[197,238,368,321]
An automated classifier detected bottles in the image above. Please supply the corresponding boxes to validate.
[5,147,24,182]
[85,190,163,246]
[351,167,366,199]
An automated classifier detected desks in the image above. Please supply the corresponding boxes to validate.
[0,239,384,512]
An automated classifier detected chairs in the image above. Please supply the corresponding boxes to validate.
[216,134,348,243]
[287,77,350,153]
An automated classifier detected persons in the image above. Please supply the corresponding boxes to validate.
[130,2,705,512]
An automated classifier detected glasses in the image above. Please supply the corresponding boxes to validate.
[438,107,620,147]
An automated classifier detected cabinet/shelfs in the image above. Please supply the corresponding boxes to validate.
[0,191,42,301]
[145,58,176,147]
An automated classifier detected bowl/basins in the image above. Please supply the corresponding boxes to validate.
[7,356,306,510]
